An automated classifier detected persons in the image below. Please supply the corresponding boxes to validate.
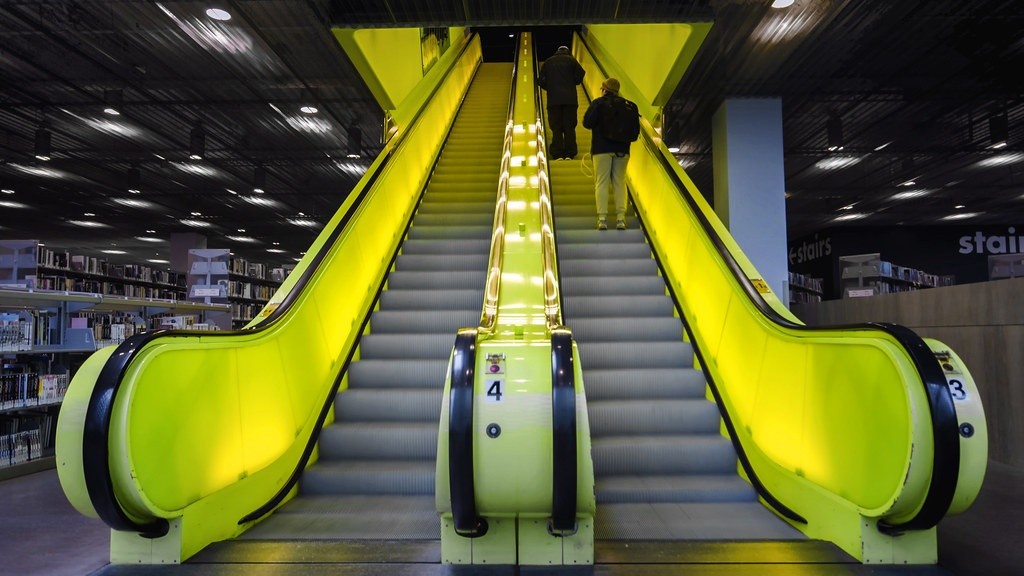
[582,78,641,230]
[538,46,586,161]
[276,270,281,279]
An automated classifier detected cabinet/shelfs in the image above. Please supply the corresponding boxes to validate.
[788,253,953,304]
[0,240,284,482]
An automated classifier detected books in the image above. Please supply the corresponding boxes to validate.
[869,261,953,296]
[0,247,221,466]
[218,257,287,318]
[789,272,821,304]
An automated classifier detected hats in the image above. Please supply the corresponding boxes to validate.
[558,46,569,52]
[600,78,620,95]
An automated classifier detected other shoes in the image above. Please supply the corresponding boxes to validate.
[599,220,607,229]
[549,143,574,160]
[616,219,625,229]
